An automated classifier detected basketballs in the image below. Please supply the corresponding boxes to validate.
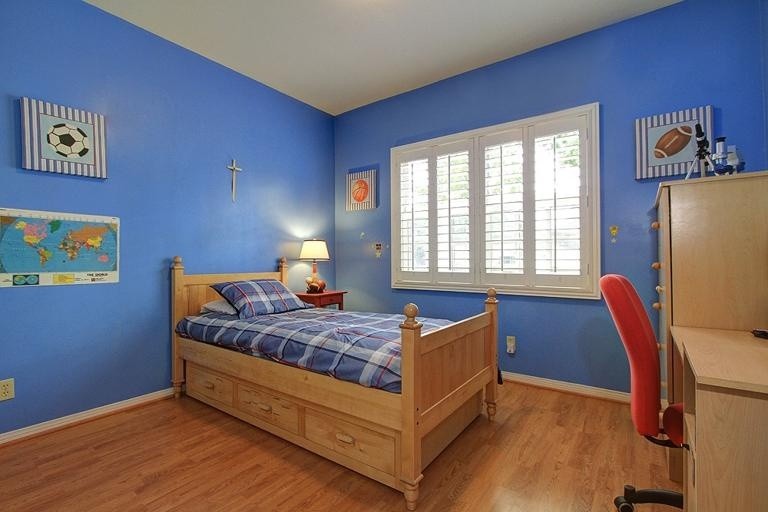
[350,179,369,202]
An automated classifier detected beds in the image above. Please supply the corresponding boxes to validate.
[169,255,499,510]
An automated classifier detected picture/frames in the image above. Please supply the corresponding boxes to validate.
[632,105,716,181]
[18,95,108,182]
[344,170,378,210]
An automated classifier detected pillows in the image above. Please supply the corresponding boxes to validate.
[200,298,238,316]
[206,279,315,319]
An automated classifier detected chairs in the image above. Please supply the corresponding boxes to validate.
[598,273,685,511]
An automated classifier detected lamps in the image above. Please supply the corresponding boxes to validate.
[299,238,330,293]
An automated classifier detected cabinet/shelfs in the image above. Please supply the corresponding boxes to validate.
[647,170,766,423]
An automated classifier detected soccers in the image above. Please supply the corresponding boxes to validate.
[47,123,91,158]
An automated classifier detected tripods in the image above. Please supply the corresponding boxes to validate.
[681,150,720,180]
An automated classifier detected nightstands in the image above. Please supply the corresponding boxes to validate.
[295,289,347,314]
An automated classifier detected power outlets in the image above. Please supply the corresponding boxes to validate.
[0,378,16,401]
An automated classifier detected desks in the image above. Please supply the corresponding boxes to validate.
[666,322,766,511]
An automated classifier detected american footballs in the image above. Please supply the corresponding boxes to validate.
[654,126,692,158]
